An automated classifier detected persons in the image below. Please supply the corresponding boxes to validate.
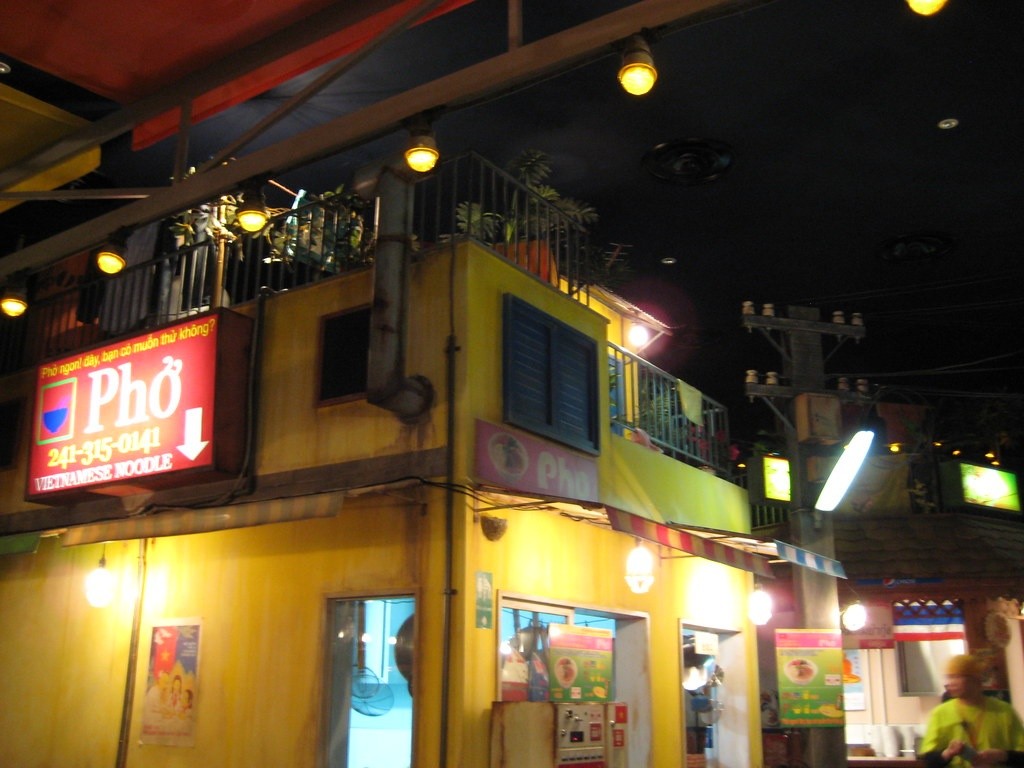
[148,673,193,713]
[919,655,1024,768]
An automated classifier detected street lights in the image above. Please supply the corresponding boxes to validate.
[788,429,875,768]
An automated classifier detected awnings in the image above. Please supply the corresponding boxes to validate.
[484,483,775,578]
[715,535,847,579]
[565,275,672,355]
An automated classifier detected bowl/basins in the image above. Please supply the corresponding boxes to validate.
[869,723,917,758]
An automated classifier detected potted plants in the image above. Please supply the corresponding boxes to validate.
[441,147,615,291]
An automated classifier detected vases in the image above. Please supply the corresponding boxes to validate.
[699,465,717,477]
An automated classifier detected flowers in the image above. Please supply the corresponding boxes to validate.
[683,423,739,464]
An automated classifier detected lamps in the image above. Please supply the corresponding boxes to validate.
[83,544,114,607]
[0,277,28,318]
[623,537,654,593]
[404,118,439,172]
[96,231,128,274]
[838,599,867,631]
[749,574,774,625]
[616,33,656,96]
[235,186,267,233]
